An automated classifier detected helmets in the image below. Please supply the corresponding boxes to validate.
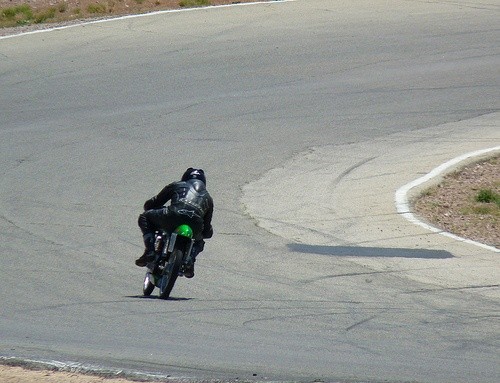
[181,168,206,185]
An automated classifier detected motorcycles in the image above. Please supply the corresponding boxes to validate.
[142,227,196,300]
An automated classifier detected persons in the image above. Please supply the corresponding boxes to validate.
[135,167,214,279]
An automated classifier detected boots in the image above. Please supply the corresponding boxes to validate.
[135,236,155,267]
[184,242,204,278]
